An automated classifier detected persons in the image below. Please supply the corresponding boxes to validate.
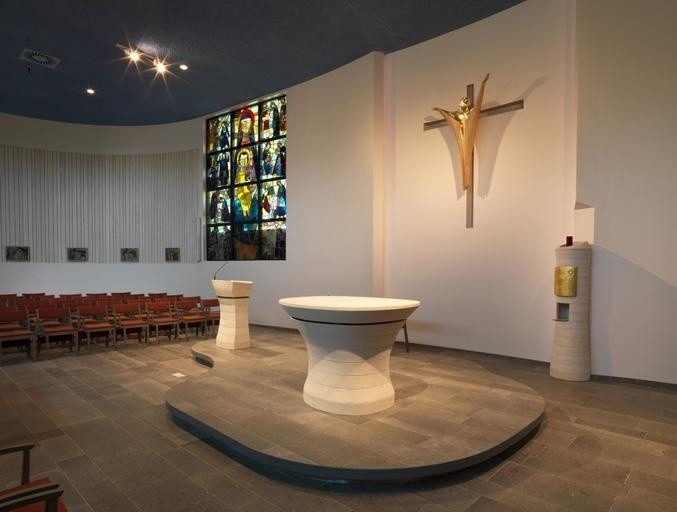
[206,100,287,260]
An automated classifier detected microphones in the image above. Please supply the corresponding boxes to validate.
[214,262,228,280]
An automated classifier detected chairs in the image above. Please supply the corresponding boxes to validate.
[0,440,64,511]
[0,292,219,361]
